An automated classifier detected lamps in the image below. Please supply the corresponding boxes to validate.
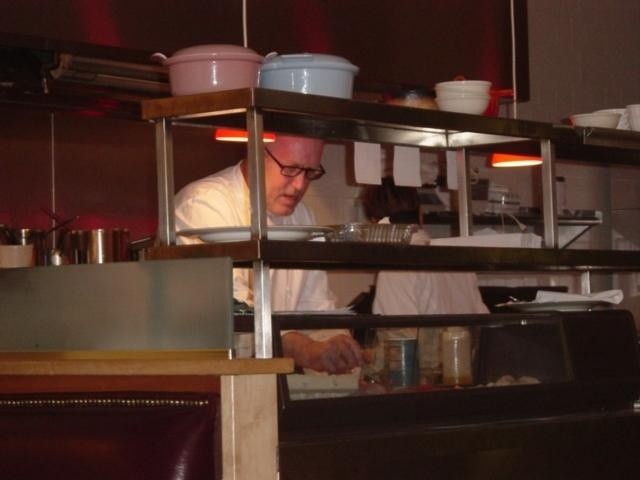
[488,1,545,168]
[213,0,277,145]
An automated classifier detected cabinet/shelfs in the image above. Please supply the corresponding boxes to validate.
[141,87,640,407]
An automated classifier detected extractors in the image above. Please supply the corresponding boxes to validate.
[454,74,514,118]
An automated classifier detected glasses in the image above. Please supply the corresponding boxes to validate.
[264,146,325,180]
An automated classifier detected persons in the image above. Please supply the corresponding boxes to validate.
[172,136,370,375]
[355,177,492,370]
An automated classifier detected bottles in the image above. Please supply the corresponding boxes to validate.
[555,173,569,211]
[439,326,475,388]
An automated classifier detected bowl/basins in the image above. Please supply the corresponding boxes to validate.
[433,79,493,117]
[570,111,622,130]
[0,244,34,268]
[38,244,63,267]
[594,108,630,131]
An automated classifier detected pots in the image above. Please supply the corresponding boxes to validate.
[59,226,155,266]
[151,43,279,98]
[0,215,77,239]
[260,50,360,100]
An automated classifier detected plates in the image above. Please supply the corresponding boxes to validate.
[174,225,337,243]
[494,300,609,314]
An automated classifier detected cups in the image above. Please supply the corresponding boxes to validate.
[625,102,640,132]
[382,337,421,389]
